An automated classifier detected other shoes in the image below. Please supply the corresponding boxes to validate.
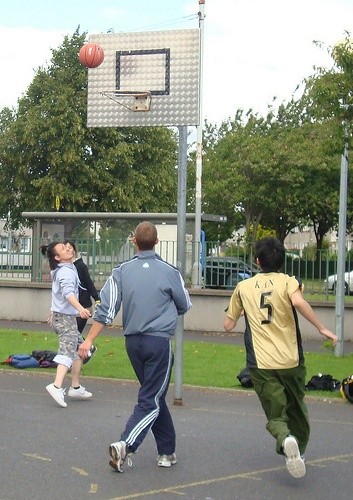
[281,435,306,478]
[83,346,97,365]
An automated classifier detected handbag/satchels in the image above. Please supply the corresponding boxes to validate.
[304,375,340,391]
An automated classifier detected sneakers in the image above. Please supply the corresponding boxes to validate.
[46,383,67,407]
[108,441,132,472]
[68,385,92,398]
[156,453,176,466]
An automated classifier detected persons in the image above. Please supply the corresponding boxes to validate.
[78,221,193,473]
[62,239,101,364]
[40,242,93,407]
[223,236,339,479]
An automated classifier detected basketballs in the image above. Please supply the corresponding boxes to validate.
[79,43,104,68]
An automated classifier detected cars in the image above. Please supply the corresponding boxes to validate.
[323,269,353,296]
[199,255,261,290]
